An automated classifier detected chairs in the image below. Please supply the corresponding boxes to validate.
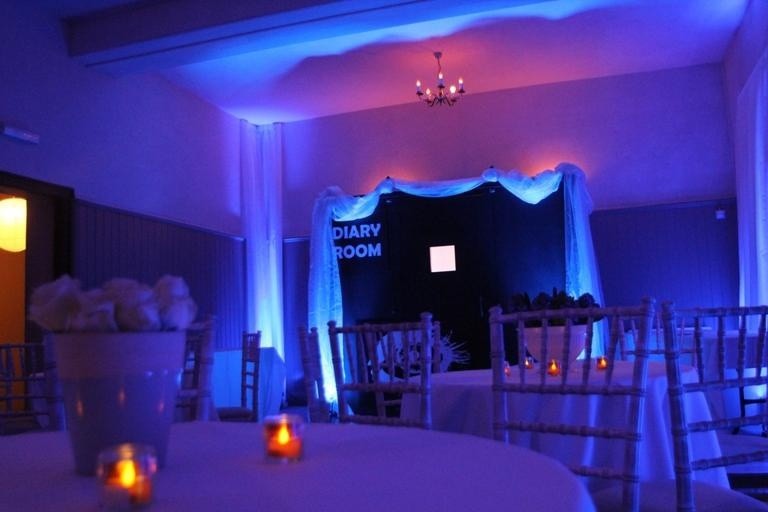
[0,327,277,423]
[292,300,768,510]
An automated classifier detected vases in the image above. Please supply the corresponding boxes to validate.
[51,328,192,475]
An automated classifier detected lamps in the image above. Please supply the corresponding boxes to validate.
[415,51,465,108]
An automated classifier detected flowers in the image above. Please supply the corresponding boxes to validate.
[27,273,202,337]
